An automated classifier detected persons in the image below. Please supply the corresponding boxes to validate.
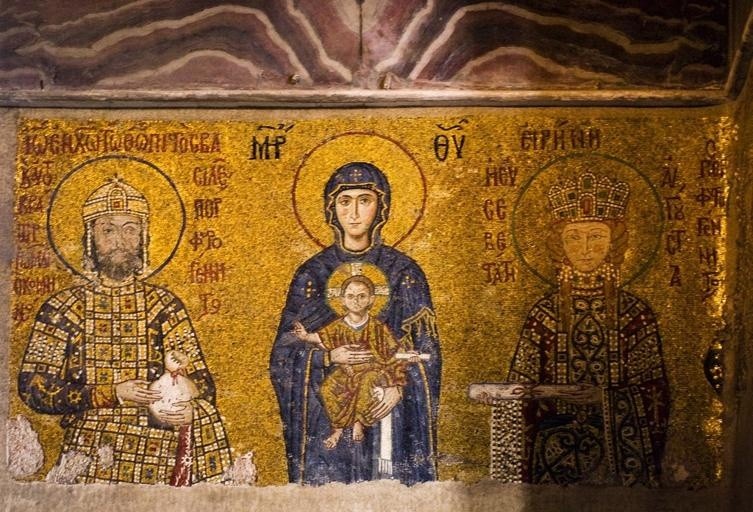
[268,163,442,485]
[289,273,420,449]
[15,172,234,485]
[463,172,669,485]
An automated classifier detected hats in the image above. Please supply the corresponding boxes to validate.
[81,172,151,224]
[546,171,631,224]
